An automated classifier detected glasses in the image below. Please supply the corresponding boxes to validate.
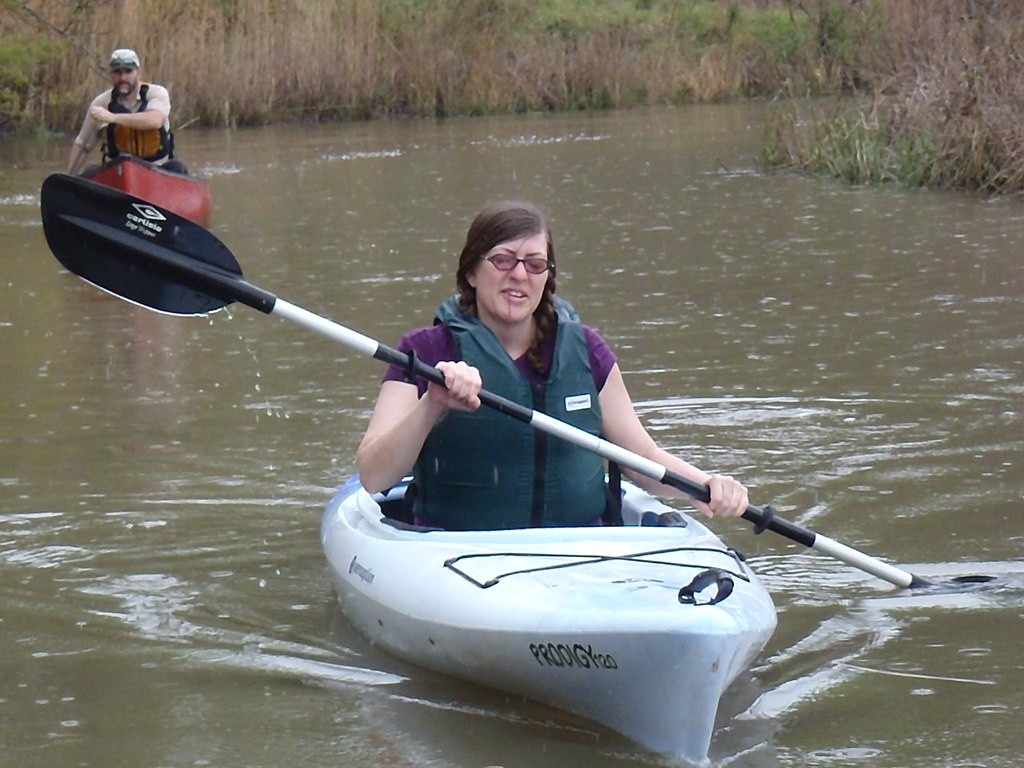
[478,254,556,274]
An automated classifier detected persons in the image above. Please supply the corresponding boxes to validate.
[359,199,747,531]
[69,49,187,176]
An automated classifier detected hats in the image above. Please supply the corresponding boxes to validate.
[107,48,140,73]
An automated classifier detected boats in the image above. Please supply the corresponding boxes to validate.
[77,152,212,230]
[318,473,780,768]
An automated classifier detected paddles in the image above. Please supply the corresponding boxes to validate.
[37,166,1003,591]
[65,117,101,175]
